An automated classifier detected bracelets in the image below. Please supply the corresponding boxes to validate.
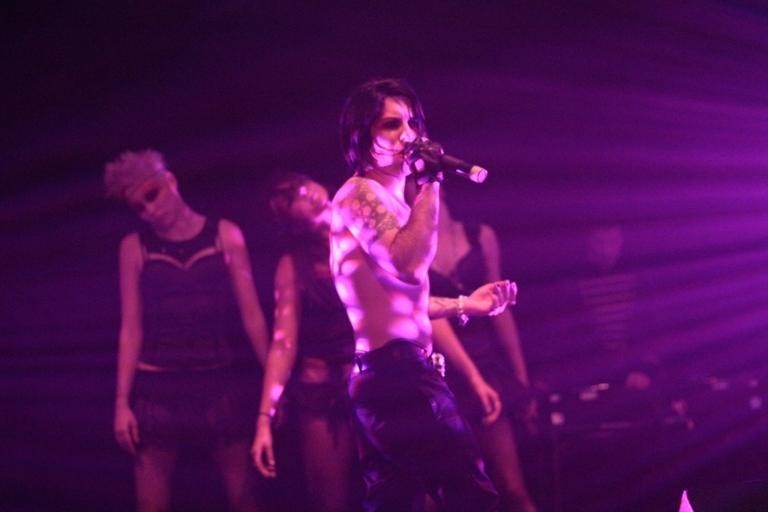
[257,411,273,419]
[457,294,470,326]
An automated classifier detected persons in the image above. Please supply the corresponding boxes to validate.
[247,154,503,511]
[103,148,288,512]
[401,164,543,511]
[325,76,524,511]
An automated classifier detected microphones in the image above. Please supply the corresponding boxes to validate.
[405,144,488,184]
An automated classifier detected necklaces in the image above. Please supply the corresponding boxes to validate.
[438,222,465,291]
